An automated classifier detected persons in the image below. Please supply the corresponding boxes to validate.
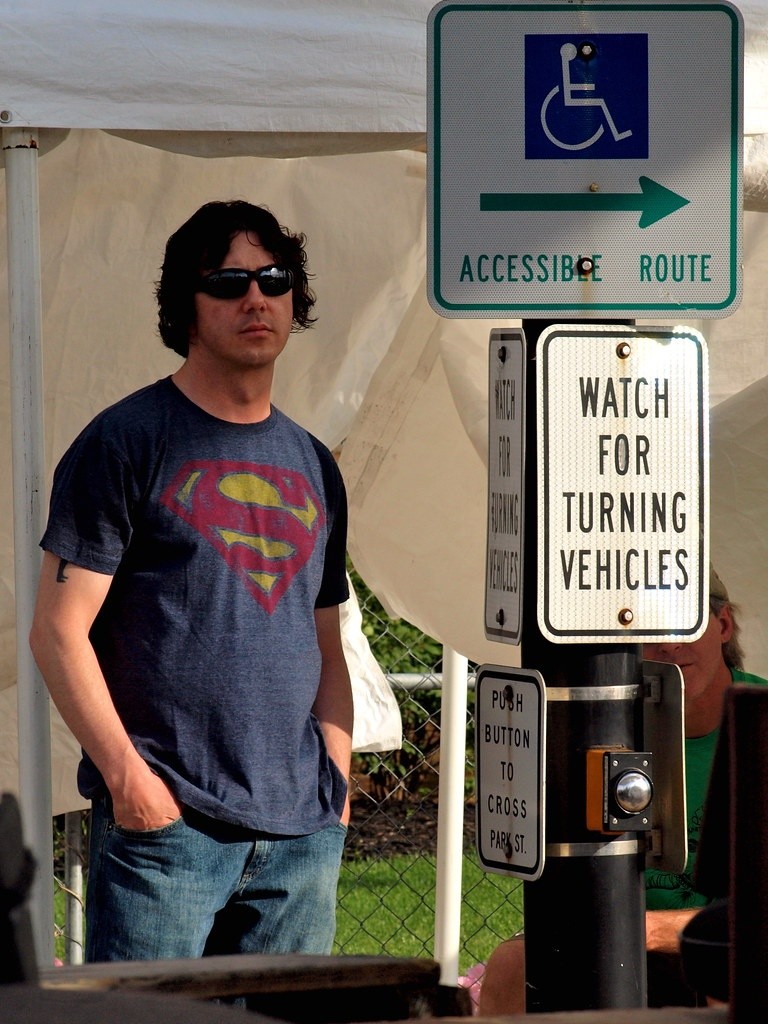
[479,562,768,1014]
[29,202,354,1018]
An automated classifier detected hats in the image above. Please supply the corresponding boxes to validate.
[711,559,729,602]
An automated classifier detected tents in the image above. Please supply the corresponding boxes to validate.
[0,0,768,963]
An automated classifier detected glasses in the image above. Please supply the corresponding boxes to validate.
[199,262,296,300]
